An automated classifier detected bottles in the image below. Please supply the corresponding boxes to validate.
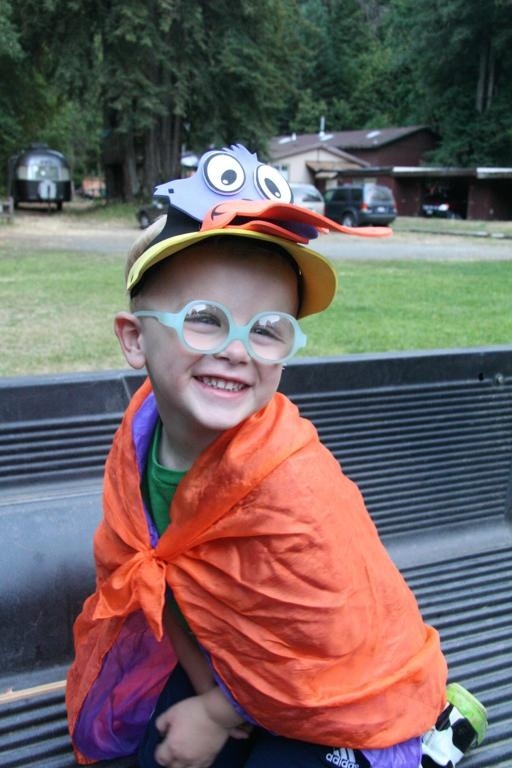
[125,143,392,314]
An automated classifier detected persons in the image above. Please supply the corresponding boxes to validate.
[67,143,488,767]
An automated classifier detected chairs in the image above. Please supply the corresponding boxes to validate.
[420,684,488,768]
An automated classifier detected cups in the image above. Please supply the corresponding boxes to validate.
[130,298,309,363]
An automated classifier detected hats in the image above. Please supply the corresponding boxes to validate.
[422,191,467,219]
[135,192,170,228]
[289,182,327,217]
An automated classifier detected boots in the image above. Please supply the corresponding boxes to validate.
[323,183,397,226]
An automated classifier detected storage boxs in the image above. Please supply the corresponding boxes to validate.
[0,338,510,766]
